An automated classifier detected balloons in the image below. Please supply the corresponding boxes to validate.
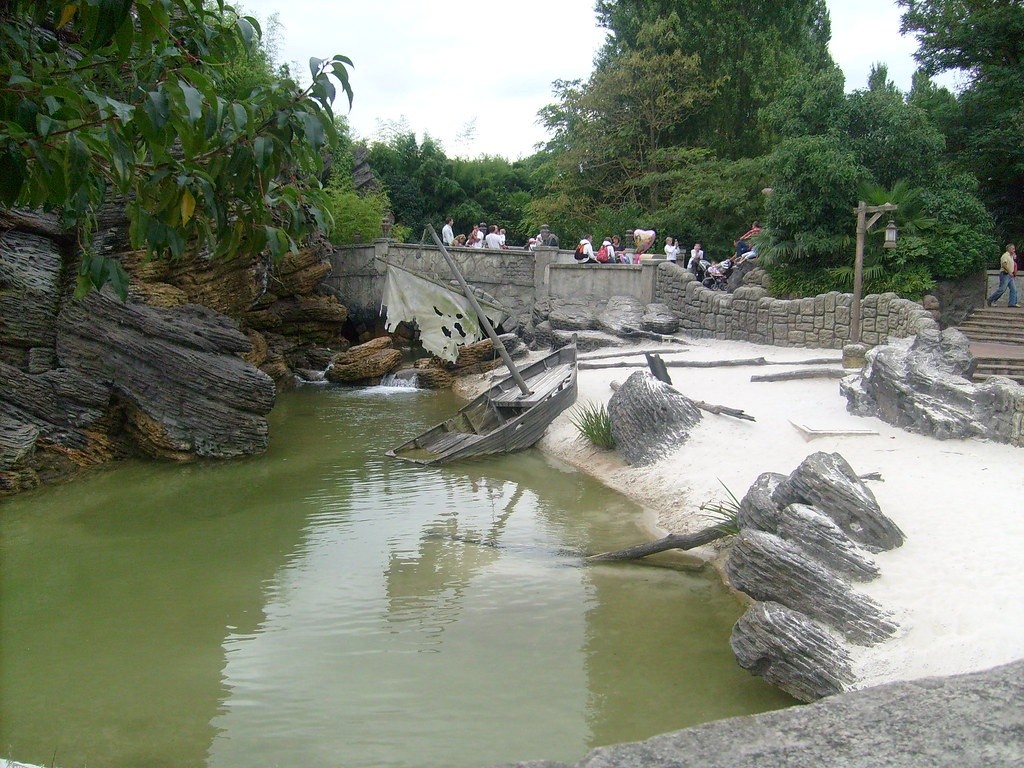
[634,229,656,253]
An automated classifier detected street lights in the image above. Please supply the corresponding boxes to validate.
[848,199,899,344]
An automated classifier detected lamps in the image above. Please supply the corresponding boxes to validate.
[883,221,896,248]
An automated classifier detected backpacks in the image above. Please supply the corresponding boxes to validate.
[597,245,611,261]
[574,243,589,260]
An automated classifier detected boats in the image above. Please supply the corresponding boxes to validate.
[385,224,579,468]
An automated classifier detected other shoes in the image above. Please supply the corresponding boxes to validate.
[987,299,991,306]
[991,302,998,307]
[1008,304,1021,308]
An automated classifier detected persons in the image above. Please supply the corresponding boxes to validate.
[686,243,720,280]
[576,233,601,264]
[485,224,506,249]
[664,237,681,264]
[986,243,1021,308]
[732,221,765,265]
[529,229,559,251]
[442,216,454,246]
[598,237,616,263]
[465,223,484,248]
[611,234,627,264]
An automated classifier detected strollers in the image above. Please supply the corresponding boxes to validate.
[698,259,728,292]
